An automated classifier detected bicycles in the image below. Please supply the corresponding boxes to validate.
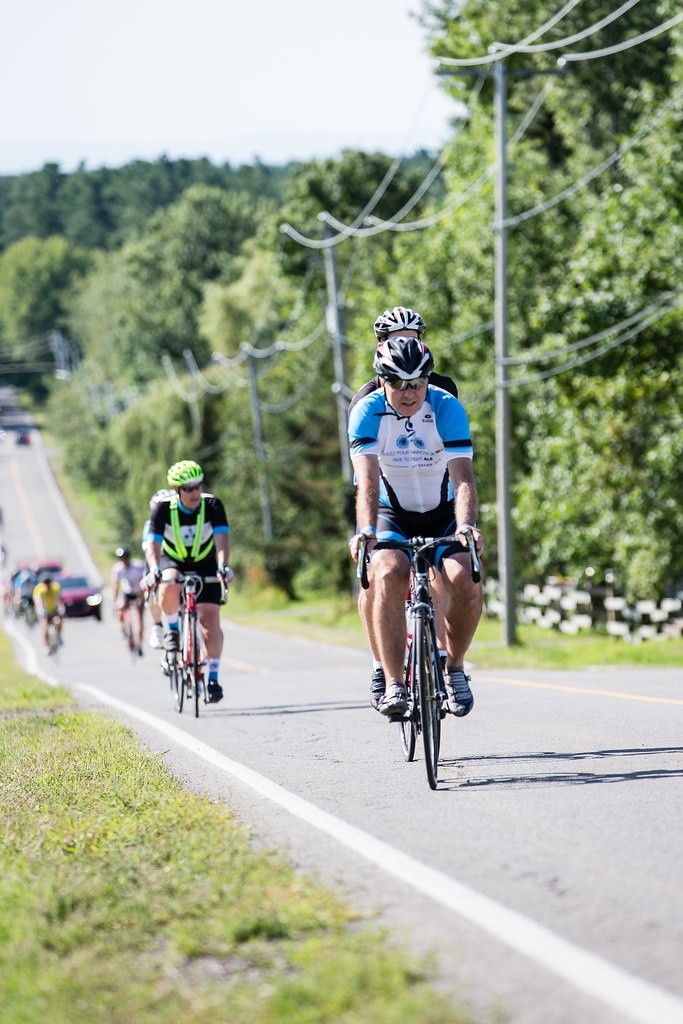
[42,611,60,656]
[115,599,140,656]
[356,530,480,791]
[145,567,228,717]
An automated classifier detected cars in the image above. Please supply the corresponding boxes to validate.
[56,579,102,621]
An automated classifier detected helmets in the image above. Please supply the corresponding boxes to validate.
[372,307,425,339]
[149,489,176,510]
[167,459,204,490]
[373,334,436,381]
[115,547,131,560]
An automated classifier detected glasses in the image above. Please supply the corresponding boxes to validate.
[388,377,428,390]
[183,482,203,493]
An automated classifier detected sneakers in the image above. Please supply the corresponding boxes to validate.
[208,681,224,703]
[371,668,386,712]
[149,623,164,648]
[380,681,408,716]
[443,668,473,718]
[162,626,179,651]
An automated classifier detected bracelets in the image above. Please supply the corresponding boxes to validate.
[360,526,376,533]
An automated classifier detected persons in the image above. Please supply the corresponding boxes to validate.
[108,547,148,653]
[345,307,458,711]
[141,488,202,650]
[3,562,65,654]
[140,460,234,703]
[347,336,484,717]
